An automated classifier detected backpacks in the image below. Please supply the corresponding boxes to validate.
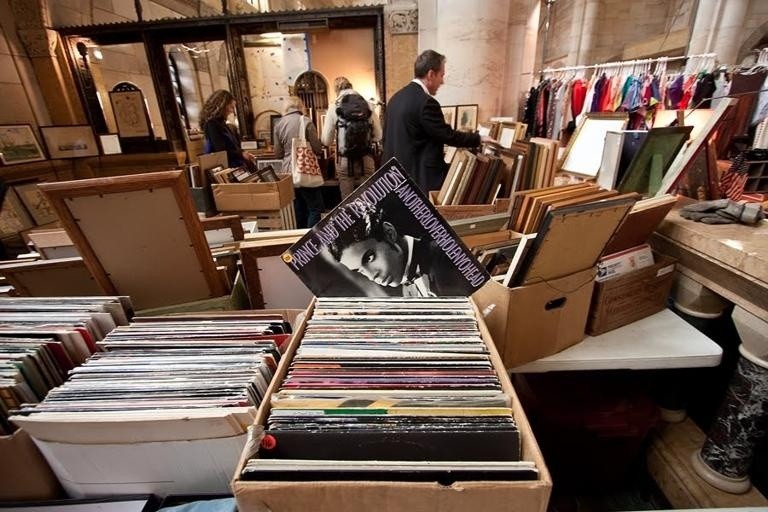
[334,92,374,164]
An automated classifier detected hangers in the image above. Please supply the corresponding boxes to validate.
[540,48,768,85]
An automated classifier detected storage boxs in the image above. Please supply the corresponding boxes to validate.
[0,172,734,512]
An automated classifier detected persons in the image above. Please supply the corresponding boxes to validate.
[320,76,384,206]
[379,49,497,199]
[325,211,473,307]
[198,89,257,175]
[271,95,324,230]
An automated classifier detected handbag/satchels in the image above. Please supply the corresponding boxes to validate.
[290,115,326,189]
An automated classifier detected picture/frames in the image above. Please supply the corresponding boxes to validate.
[0,82,740,312]
